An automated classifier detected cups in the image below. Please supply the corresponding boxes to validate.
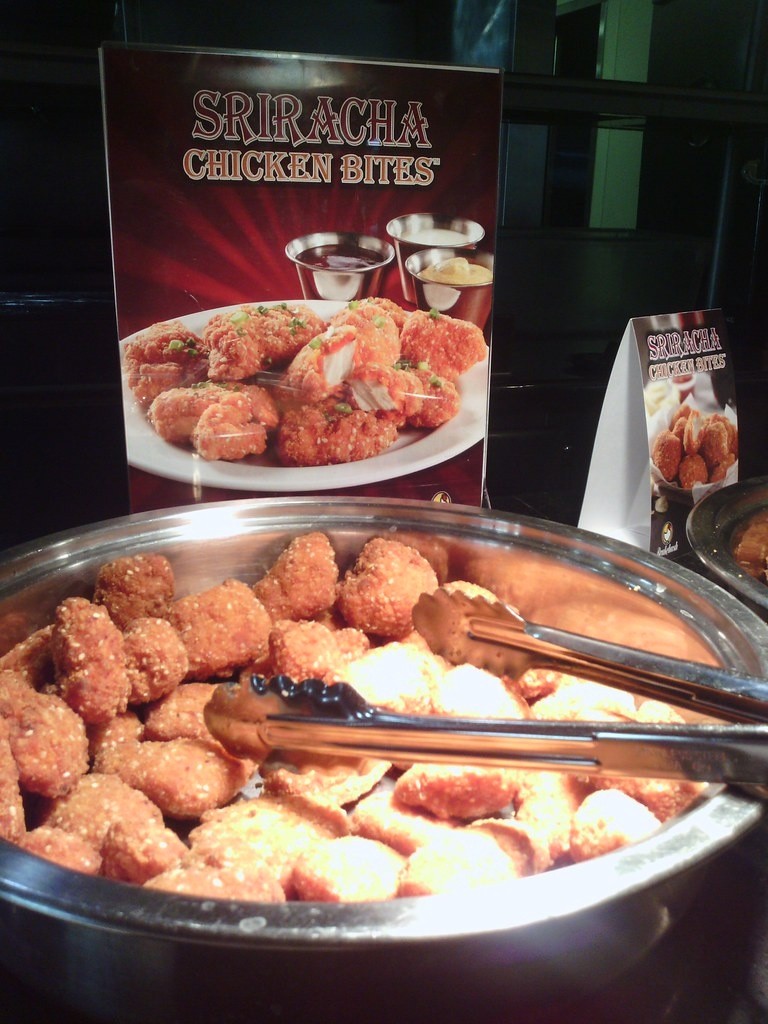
[285,231,396,301]
[405,247,493,334]
[669,374,697,404]
[386,212,485,312]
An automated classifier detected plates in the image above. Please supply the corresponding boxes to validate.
[119,300,490,492]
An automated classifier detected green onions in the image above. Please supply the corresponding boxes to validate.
[165,297,447,413]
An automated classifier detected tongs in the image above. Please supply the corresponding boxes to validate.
[203,588,768,786]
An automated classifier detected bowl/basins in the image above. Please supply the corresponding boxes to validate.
[684,478,768,616]
[0,493,767,1024]
